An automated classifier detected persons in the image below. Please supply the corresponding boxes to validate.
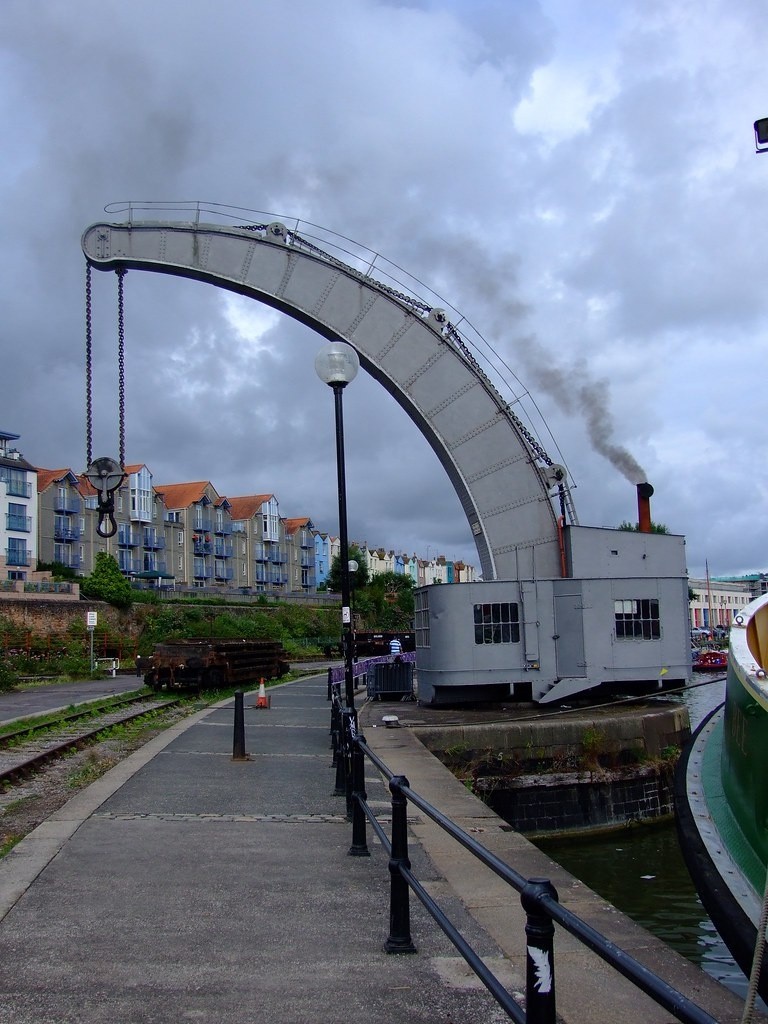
[135,655,141,677]
[389,636,403,655]
[709,643,723,664]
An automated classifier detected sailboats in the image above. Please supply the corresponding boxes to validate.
[694,557,728,669]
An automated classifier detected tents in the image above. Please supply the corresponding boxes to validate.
[134,570,176,591]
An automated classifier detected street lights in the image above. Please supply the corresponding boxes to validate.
[313,338,359,735]
[348,560,358,689]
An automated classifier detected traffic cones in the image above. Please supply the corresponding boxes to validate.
[255,678,270,708]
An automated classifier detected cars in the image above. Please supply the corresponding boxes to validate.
[690,627,711,637]
[702,627,726,638]
